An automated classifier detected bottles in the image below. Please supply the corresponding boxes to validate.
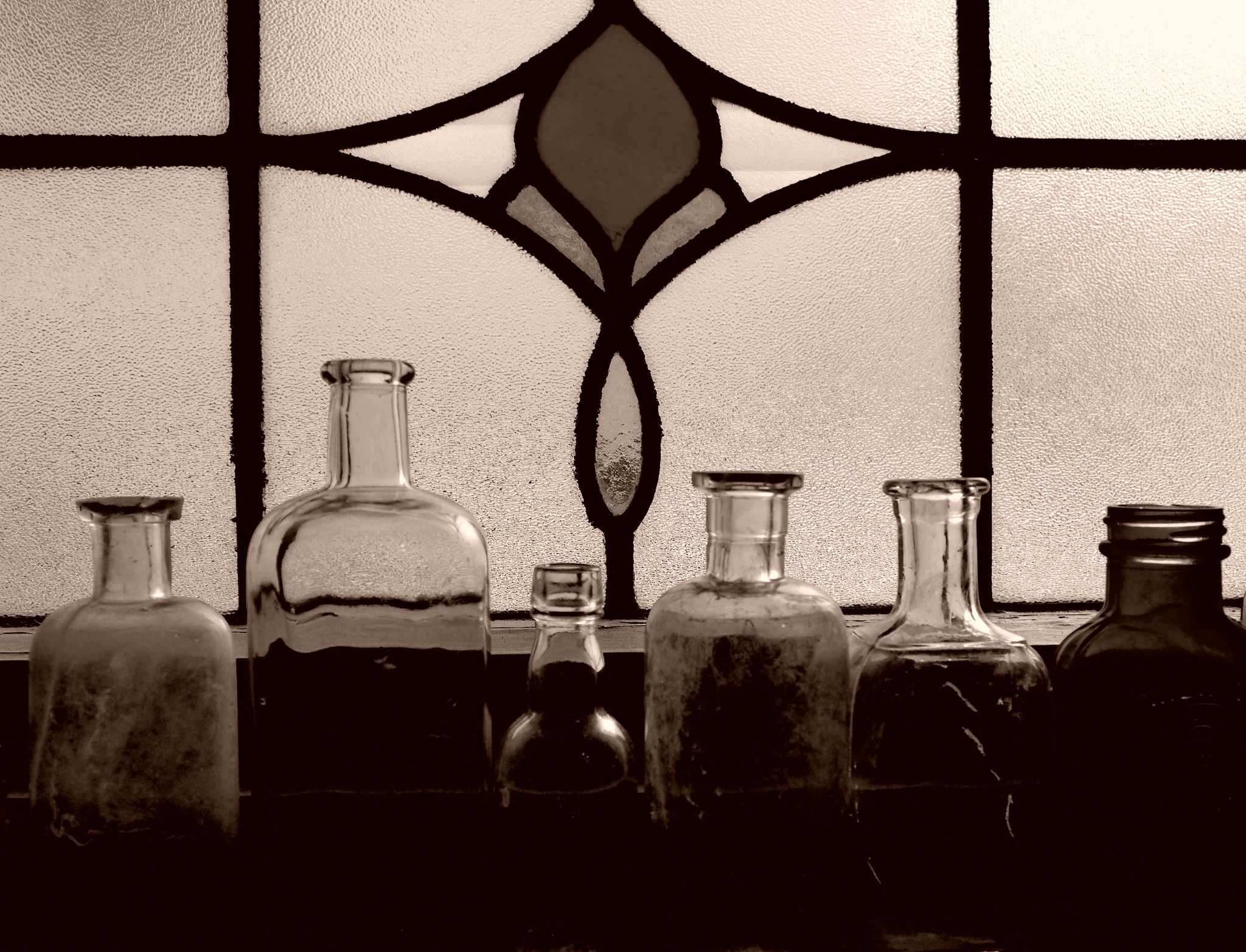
[236,356,495,792]
[1055,504,1245,730]
[849,477,1058,891]
[493,563,634,819]
[641,468,851,819]
[19,498,240,858]
